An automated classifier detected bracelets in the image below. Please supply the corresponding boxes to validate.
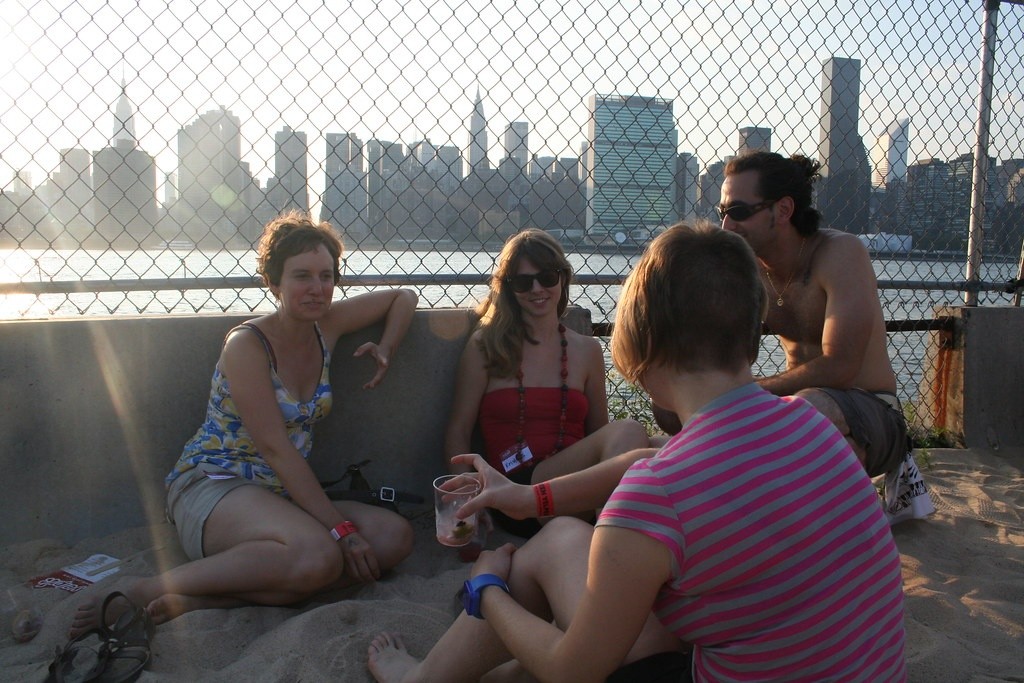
[329,520,358,543]
[534,481,555,522]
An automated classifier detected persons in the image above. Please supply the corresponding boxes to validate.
[68,220,419,637]
[445,228,673,543]
[367,224,905,682]
[652,153,912,480]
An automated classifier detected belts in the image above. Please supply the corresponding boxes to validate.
[876,393,902,411]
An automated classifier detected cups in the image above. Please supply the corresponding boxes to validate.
[432,474,481,547]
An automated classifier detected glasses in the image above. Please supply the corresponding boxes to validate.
[715,195,786,222]
[508,269,560,293]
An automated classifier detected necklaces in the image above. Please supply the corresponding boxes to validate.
[516,322,568,469]
[766,234,805,306]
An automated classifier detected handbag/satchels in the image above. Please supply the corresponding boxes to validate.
[322,461,424,513]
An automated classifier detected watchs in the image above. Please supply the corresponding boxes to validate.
[461,573,510,620]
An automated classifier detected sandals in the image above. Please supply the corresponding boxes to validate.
[100,591,155,683]
[43,625,110,683]
